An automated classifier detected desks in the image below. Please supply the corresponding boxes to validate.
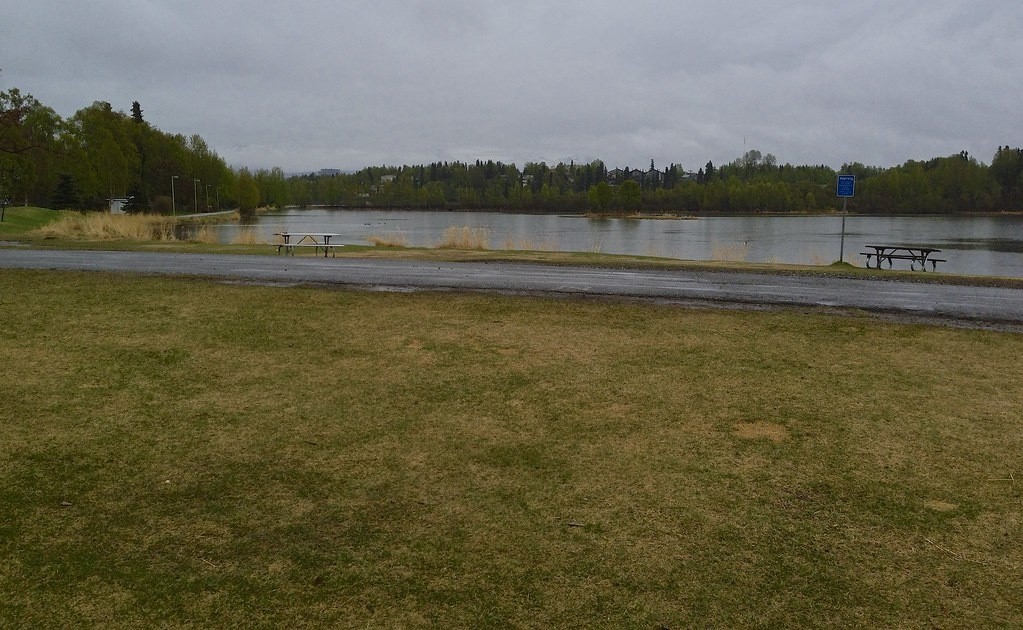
[273,232,342,257]
[865,245,941,271]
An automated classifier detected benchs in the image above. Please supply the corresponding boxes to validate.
[860,253,947,272]
[270,242,345,258]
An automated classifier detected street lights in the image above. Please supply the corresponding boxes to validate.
[217,186,221,210]
[194,178,200,213]
[172,176,178,217]
[206,184,211,213]
